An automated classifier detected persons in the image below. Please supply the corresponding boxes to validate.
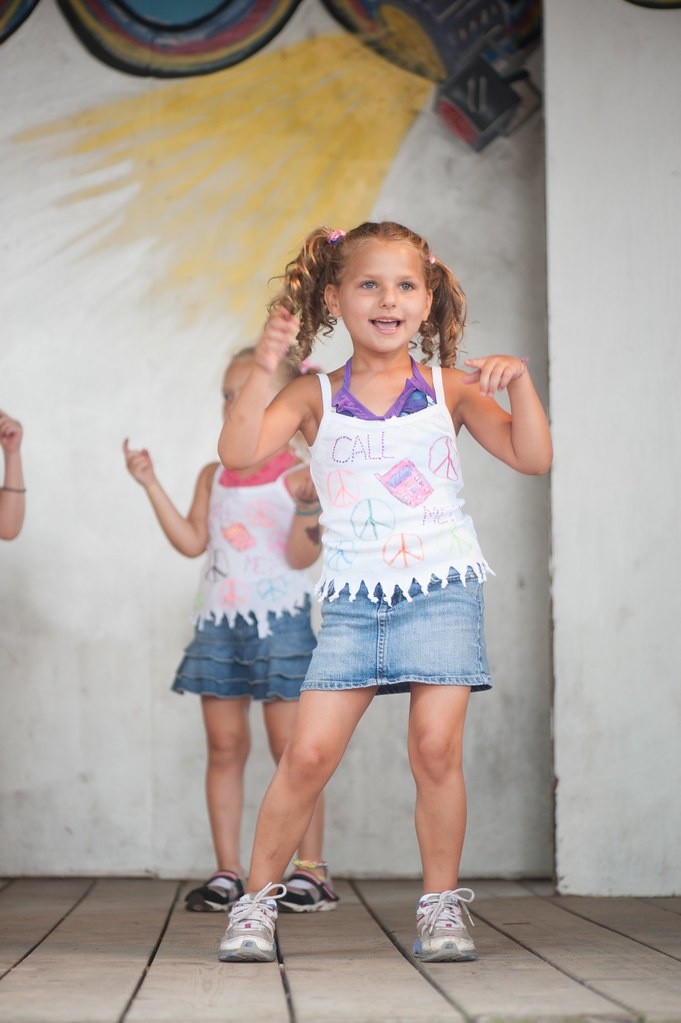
[124,346,342,910]
[0,411,27,540]
[219,223,553,964]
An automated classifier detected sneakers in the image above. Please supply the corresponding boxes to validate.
[413,887,478,962]
[219,882,287,962]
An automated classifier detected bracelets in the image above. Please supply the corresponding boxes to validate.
[3,484,26,494]
[294,506,320,518]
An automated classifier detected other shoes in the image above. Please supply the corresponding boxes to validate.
[185,871,246,912]
[277,868,339,912]
